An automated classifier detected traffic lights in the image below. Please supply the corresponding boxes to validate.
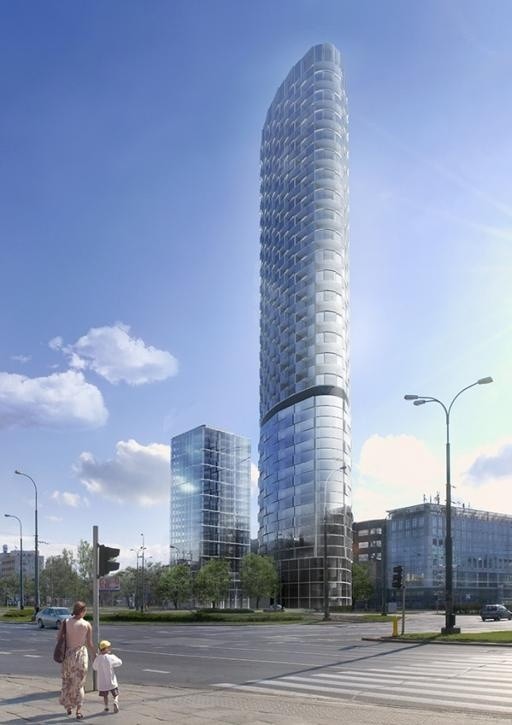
[391,565,405,589]
[98,543,120,577]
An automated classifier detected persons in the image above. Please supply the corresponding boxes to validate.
[52,600,97,721]
[90,639,124,715]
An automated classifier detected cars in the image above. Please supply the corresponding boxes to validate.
[35,606,73,630]
[479,604,511,621]
[263,605,287,612]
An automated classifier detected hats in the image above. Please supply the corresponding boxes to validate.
[99,640,111,650]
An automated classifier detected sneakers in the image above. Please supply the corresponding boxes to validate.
[113,702,119,713]
[104,708,109,711]
[67,711,72,715]
[77,713,81,719]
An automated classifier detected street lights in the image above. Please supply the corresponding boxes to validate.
[403,376,495,635]
[322,465,348,621]
[3,469,40,621]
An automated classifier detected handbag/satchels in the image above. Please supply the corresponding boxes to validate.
[53,618,67,663]
